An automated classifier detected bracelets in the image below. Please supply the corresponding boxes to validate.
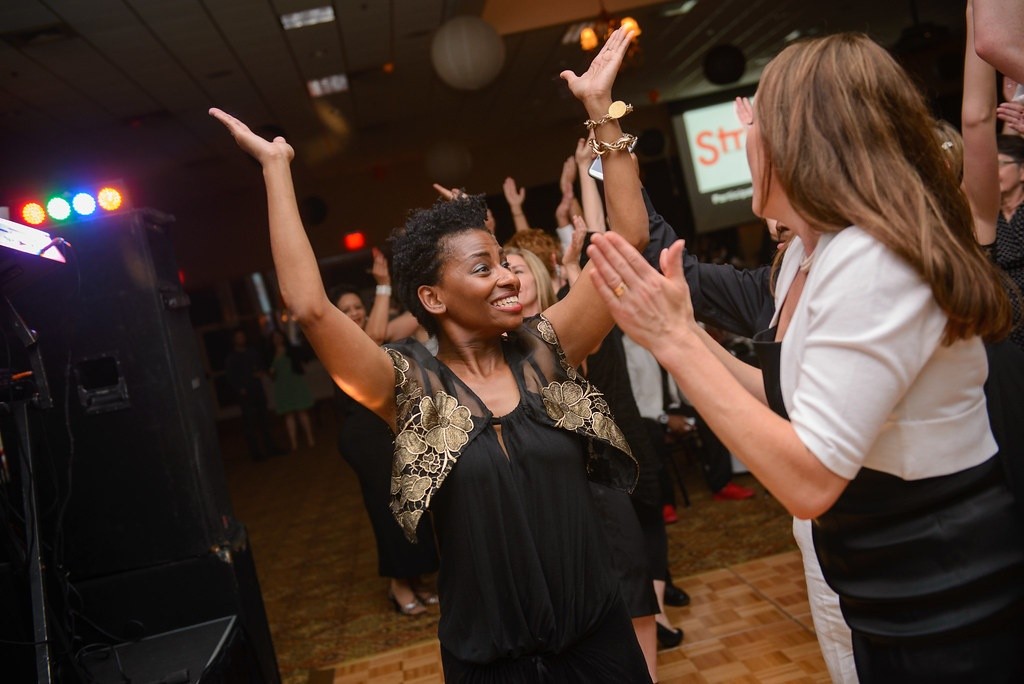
[512,212,525,217]
[588,133,638,156]
[583,101,633,130]
[376,285,393,296]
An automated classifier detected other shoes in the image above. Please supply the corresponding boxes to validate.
[663,584,689,606]
[712,480,755,501]
[662,504,678,525]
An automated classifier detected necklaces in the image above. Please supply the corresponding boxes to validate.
[799,252,815,272]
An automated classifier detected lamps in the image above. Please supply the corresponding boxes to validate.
[578,0,641,54]
[429,16,509,90]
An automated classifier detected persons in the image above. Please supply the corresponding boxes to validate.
[631,1,1024,683]
[206,27,762,684]
[586,33,1024,682]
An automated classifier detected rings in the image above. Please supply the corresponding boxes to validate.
[615,283,627,297]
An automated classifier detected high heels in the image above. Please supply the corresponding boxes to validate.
[657,621,682,644]
[388,588,426,615]
[411,582,439,604]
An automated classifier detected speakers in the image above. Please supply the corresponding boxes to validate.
[0,208,286,684]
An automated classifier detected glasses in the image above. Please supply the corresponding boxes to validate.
[998,159,1024,168]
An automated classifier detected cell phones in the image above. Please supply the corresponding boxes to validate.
[588,139,638,181]
